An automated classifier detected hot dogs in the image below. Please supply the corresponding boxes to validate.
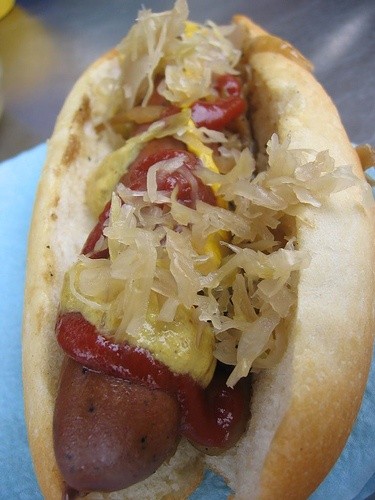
[21,1,375,495]
